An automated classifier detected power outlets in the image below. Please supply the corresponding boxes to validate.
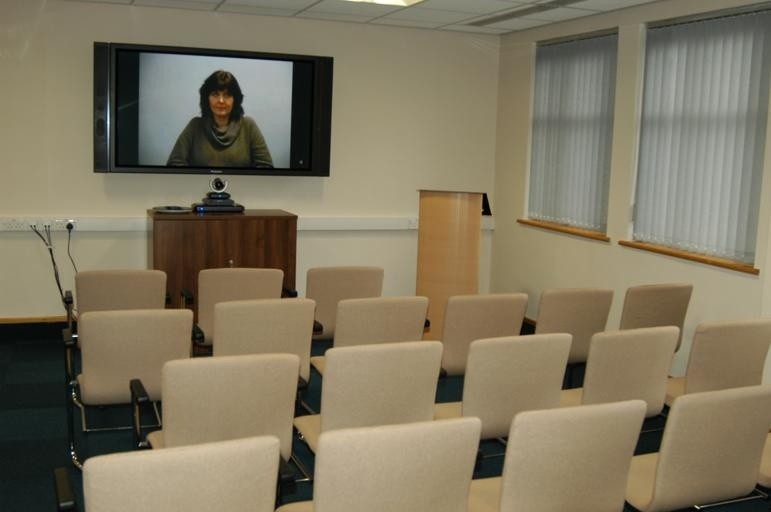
[0,219,79,233]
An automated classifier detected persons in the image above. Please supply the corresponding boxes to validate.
[166,70,273,168]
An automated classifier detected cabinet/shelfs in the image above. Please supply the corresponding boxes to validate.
[145,208,298,311]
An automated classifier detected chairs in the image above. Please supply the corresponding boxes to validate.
[664,320,771,408]
[468,400,646,510]
[307,267,383,342]
[60,308,193,473]
[620,283,695,353]
[277,416,482,510]
[129,353,311,483]
[536,287,615,368]
[560,326,680,420]
[209,297,316,419]
[424,292,529,376]
[435,334,572,446]
[65,269,167,330]
[54,436,280,512]
[300,296,430,415]
[293,339,442,482]
[180,267,298,345]
[626,385,771,511]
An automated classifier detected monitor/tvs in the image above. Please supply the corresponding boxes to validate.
[89,32,334,179]
